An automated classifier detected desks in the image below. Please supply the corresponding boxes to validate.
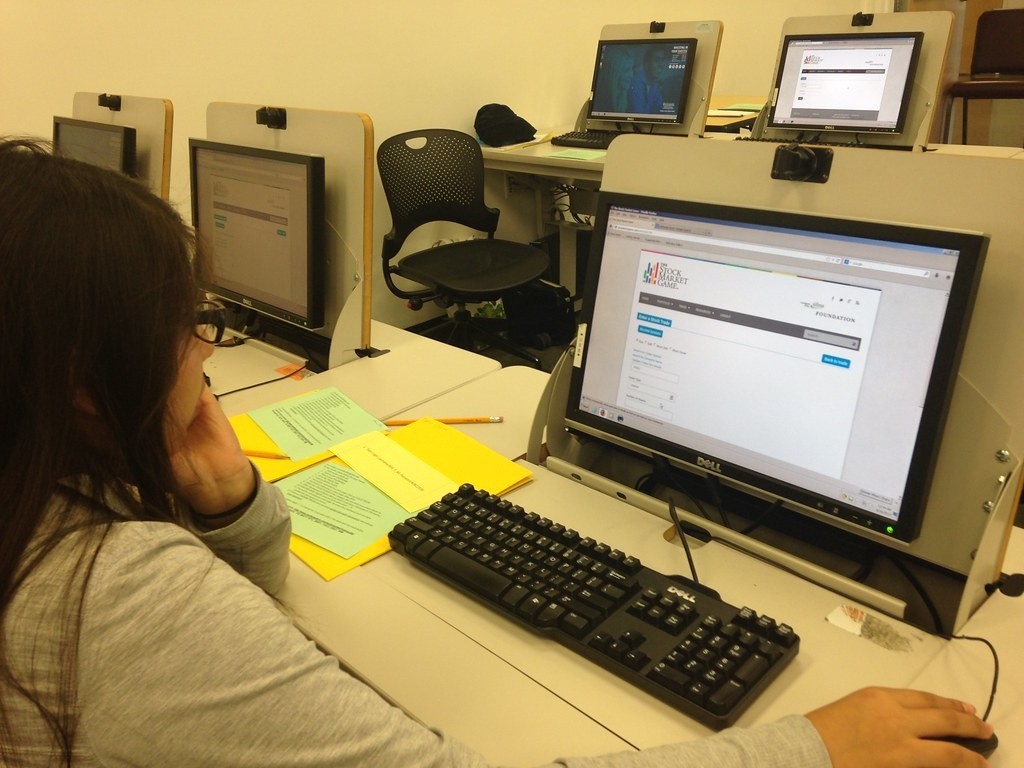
[179,312,1024,768]
[480,124,1024,321]
[705,97,767,133]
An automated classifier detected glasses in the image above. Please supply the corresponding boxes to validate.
[179,299,226,344]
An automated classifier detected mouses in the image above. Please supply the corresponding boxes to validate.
[922,729,999,762]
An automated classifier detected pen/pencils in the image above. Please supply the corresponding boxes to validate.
[243,449,291,459]
[382,416,504,425]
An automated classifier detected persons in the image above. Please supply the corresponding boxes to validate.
[626,48,666,113]
[0,139,992,768]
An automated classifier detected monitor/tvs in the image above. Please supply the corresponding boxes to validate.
[587,38,698,125]
[565,189,991,549]
[189,138,326,333]
[766,32,924,135]
[54,116,136,178]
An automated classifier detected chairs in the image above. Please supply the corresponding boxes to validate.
[377,129,572,368]
[937,8,1024,146]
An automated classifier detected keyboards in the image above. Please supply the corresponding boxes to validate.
[388,483,800,732]
[551,131,621,149]
